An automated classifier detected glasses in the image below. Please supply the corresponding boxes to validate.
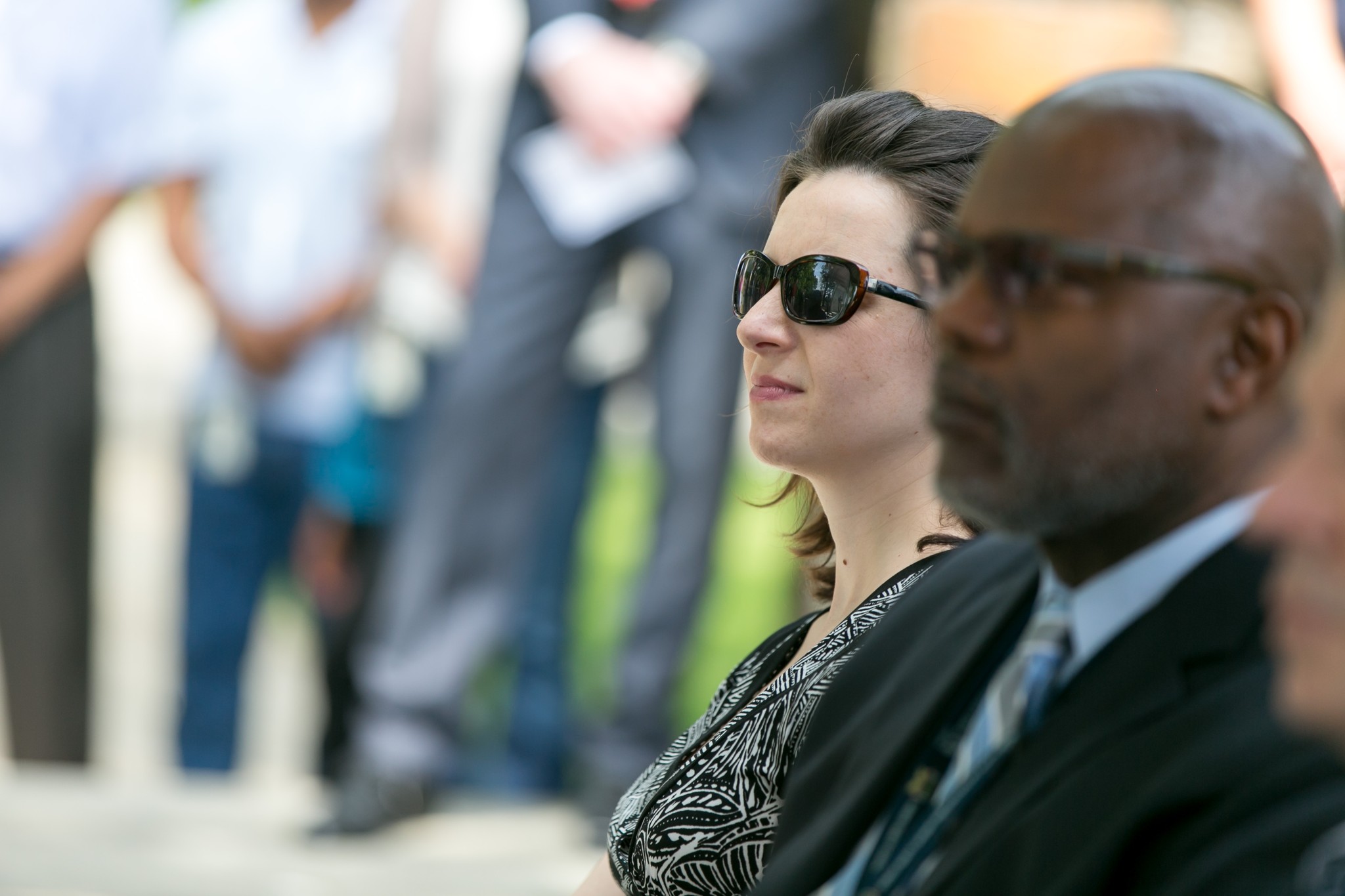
[924,232,1256,315]
[733,244,936,326]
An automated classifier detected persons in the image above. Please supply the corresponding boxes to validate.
[589,72,1345,896]
[0,0,887,841]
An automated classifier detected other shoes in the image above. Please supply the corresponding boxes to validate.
[314,765,453,838]
[581,769,635,846]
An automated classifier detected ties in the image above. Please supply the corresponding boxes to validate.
[856,588,1077,895]
